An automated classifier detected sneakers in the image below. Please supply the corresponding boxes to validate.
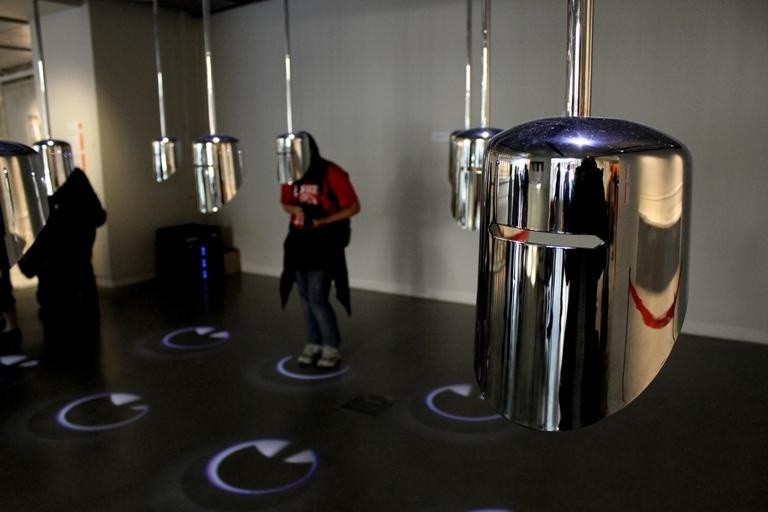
[298,343,321,366]
[317,346,341,369]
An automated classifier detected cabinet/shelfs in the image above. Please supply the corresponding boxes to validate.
[153,222,227,323]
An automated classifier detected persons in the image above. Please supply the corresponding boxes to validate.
[0,205,19,333]
[278,131,361,372]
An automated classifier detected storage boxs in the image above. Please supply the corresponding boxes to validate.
[224,248,240,276]
